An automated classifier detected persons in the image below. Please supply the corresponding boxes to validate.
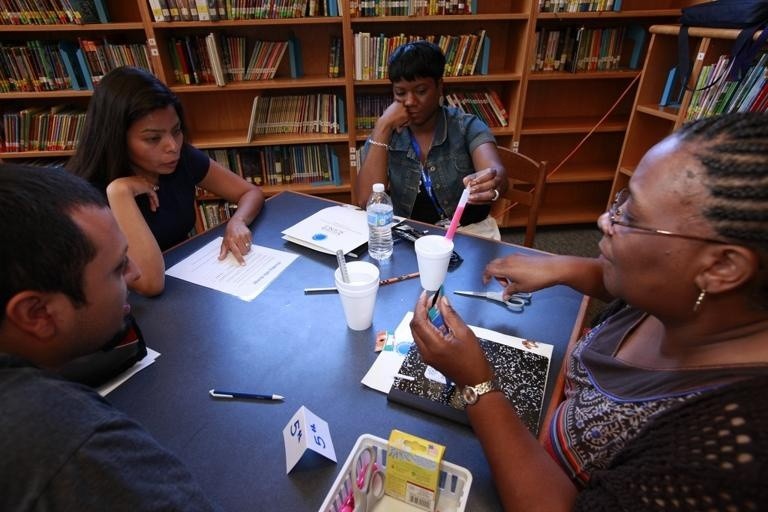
[353,39,510,242]
[64,66,265,298]
[0,160,215,511]
[407,110,768,511]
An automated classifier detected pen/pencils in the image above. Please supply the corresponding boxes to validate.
[379,270,419,287]
[304,287,337,293]
[209,389,286,402]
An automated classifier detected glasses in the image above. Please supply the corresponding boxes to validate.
[609,187,748,248]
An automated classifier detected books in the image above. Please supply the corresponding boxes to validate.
[148,0,343,23]
[385,330,550,439]
[439,87,509,129]
[281,204,408,258]
[354,92,394,130]
[684,51,768,124]
[355,144,366,176]
[195,142,342,198]
[197,201,239,232]
[0,33,154,94]
[0,101,88,154]
[348,0,478,18]
[246,93,346,143]
[167,30,306,88]
[352,28,492,81]
[328,35,344,79]
[0,0,111,26]
[537,0,623,13]
[530,22,646,74]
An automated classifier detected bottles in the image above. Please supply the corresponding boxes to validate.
[366,183,395,261]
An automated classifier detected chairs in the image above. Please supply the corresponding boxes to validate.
[493,143,549,250]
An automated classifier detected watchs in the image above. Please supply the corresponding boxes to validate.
[462,380,503,406]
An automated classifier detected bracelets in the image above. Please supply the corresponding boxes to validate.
[367,138,393,152]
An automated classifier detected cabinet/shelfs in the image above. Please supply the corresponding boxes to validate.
[0,0,683,230]
[607,26,768,213]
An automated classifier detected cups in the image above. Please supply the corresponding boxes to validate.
[414,235,454,292]
[333,260,382,332]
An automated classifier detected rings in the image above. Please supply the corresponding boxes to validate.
[492,188,500,201]
[245,243,250,247]
[152,184,160,191]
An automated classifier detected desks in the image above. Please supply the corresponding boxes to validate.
[105,190,589,512]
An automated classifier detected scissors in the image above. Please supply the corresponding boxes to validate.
[453,290,531,309]
